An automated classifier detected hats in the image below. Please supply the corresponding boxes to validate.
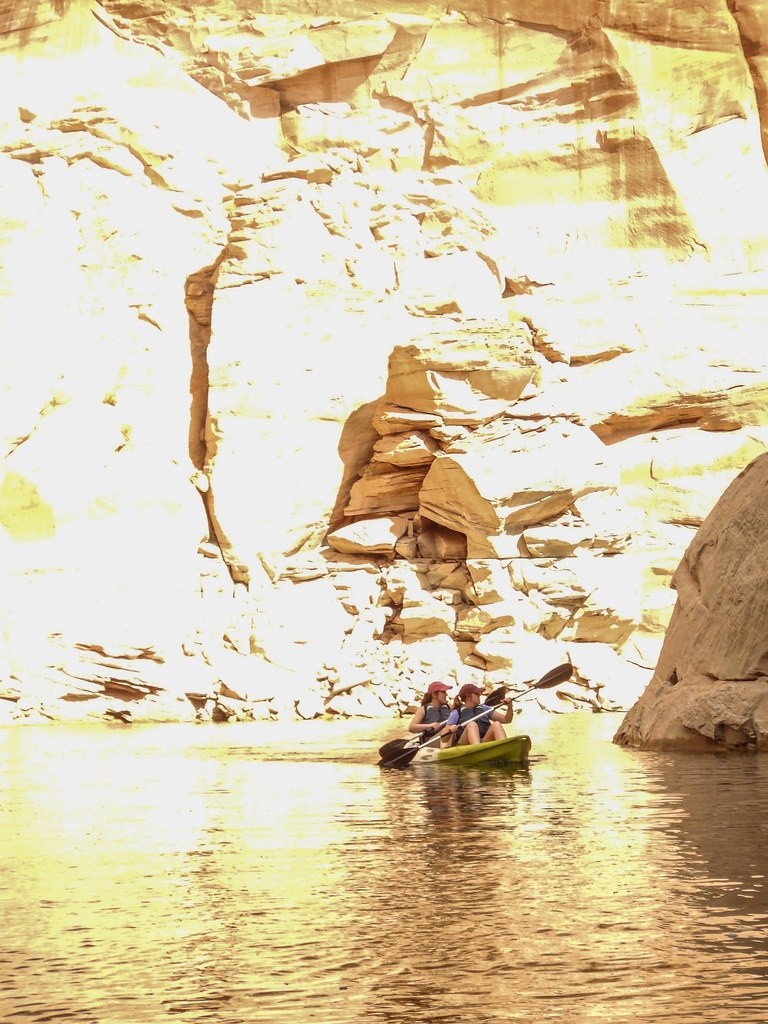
[428,681,452,694]
[460,684,486,694]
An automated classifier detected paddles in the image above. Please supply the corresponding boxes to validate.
[374,663,573,768]
[379,686,506,758]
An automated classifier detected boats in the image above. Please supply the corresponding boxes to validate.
[403,735,532,764]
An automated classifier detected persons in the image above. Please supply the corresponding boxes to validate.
[440,684,514,746]
[409,681,456,749]
